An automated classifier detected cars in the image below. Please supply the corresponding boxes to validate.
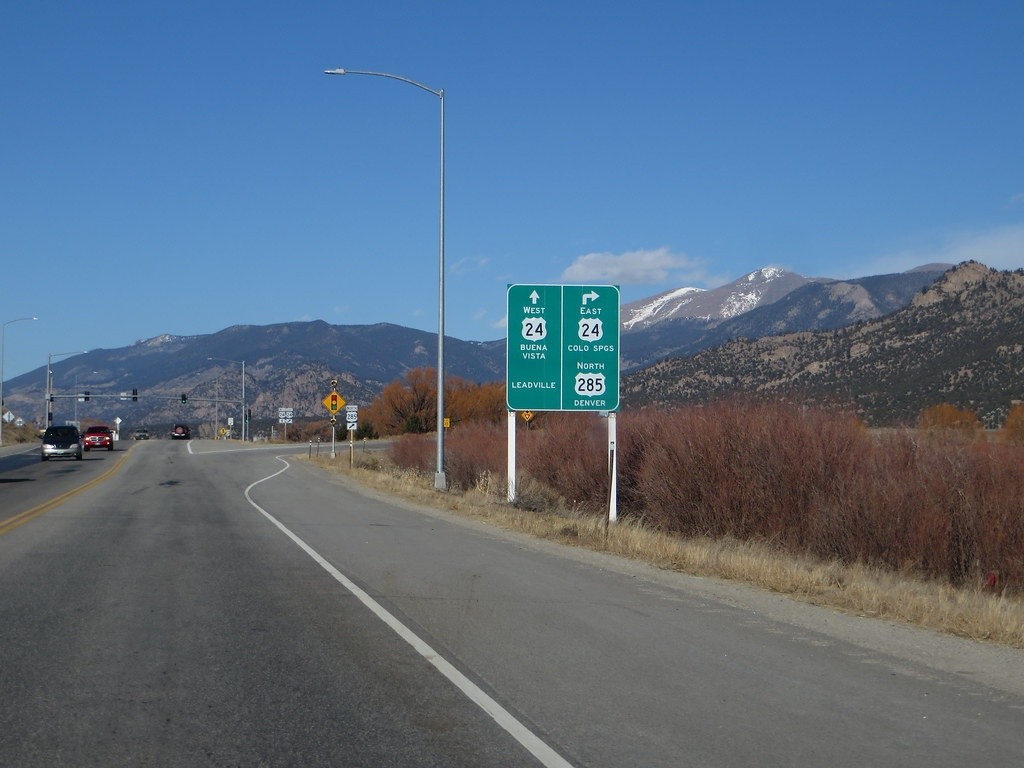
[40,423,81,460]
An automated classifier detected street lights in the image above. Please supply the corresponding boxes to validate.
[2,317,40,436]
[46,351,89,426]
[196,373,218,439]
[317,67,447,497]
[75,371,101,419]
[203,355,245,441]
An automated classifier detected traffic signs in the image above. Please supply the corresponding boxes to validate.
[507,282,614,412]
[345,405,359,430]
[278,407,294,423]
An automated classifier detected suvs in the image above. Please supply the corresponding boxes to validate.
[134,428,149,441]
[84,426,113,451]
[169,423,190,440]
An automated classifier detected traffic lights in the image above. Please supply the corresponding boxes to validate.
[181,394,186,404]
